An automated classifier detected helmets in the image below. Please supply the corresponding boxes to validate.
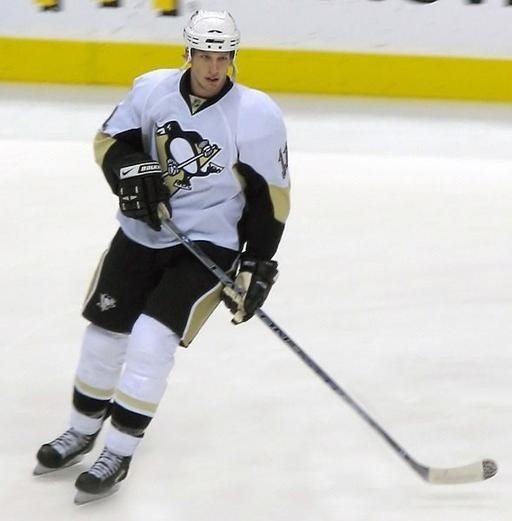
[179,7,242,64]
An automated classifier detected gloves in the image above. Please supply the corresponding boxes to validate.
[114,158,173,232]
[220,255,280,326]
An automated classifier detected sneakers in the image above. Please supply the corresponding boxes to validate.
[74,442,133,495]
[35,425,102,469]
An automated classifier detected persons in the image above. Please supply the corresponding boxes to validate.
[34,9,295,497]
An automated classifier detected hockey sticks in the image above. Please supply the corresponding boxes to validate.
[157,204,496,485]
